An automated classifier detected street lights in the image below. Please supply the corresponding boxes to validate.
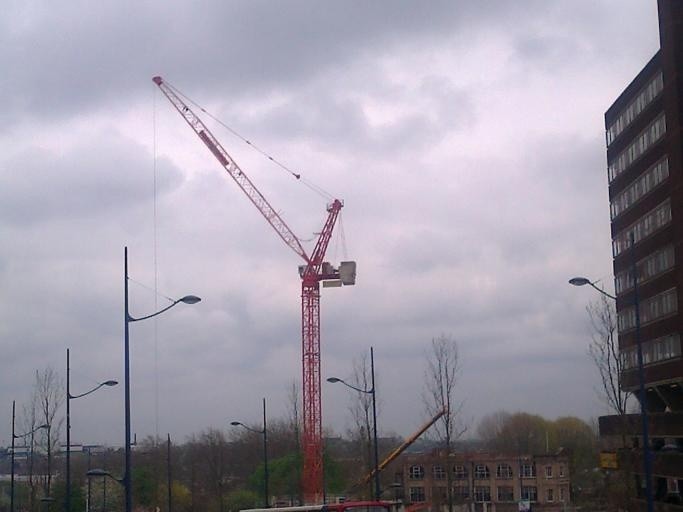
[229,394,275,511]
[39,346,119,511]
[327,344,384,511]
[87,235,205,512]
[1,395,53,509]
[569,228,656,507]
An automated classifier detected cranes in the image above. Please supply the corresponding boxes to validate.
[149,70,359,509]
